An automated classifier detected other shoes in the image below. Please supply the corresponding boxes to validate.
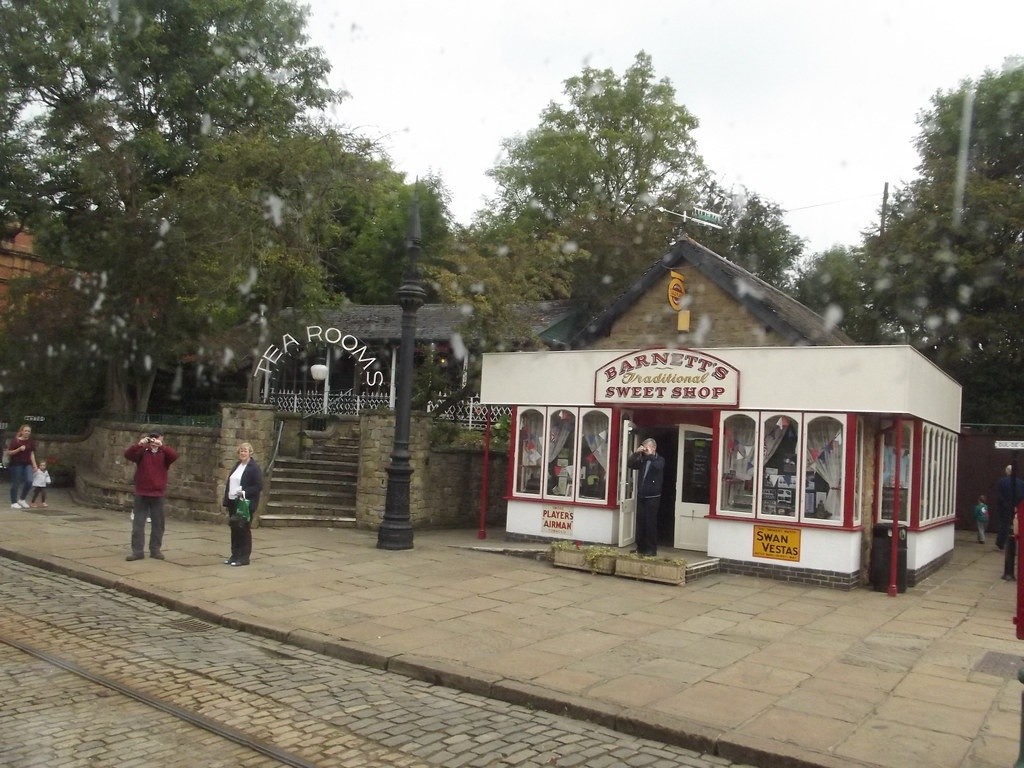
[127,554,144,561]
[31,502,38,507]
[224,557,251,567]
[630,549,656,557]
[151,550,165,559]
[41,502,48,507]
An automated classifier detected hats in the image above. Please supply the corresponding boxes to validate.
[149,428,161,437]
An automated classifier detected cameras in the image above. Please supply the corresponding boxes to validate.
[640,447,647,452]
[148,439,154,443]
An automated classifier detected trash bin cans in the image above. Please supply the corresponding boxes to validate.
[871,523,908,593]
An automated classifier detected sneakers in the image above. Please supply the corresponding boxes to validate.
[12,500,29,509]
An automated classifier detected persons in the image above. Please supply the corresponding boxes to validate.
[29,459,52,508]
[123,430,180,562]
[627,437,665,557]
[995,466,1024,550]
[975,495,990,544]
[7,425,37,510]
[221,442,264,566]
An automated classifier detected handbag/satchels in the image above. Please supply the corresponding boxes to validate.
[234,492,250,523]
